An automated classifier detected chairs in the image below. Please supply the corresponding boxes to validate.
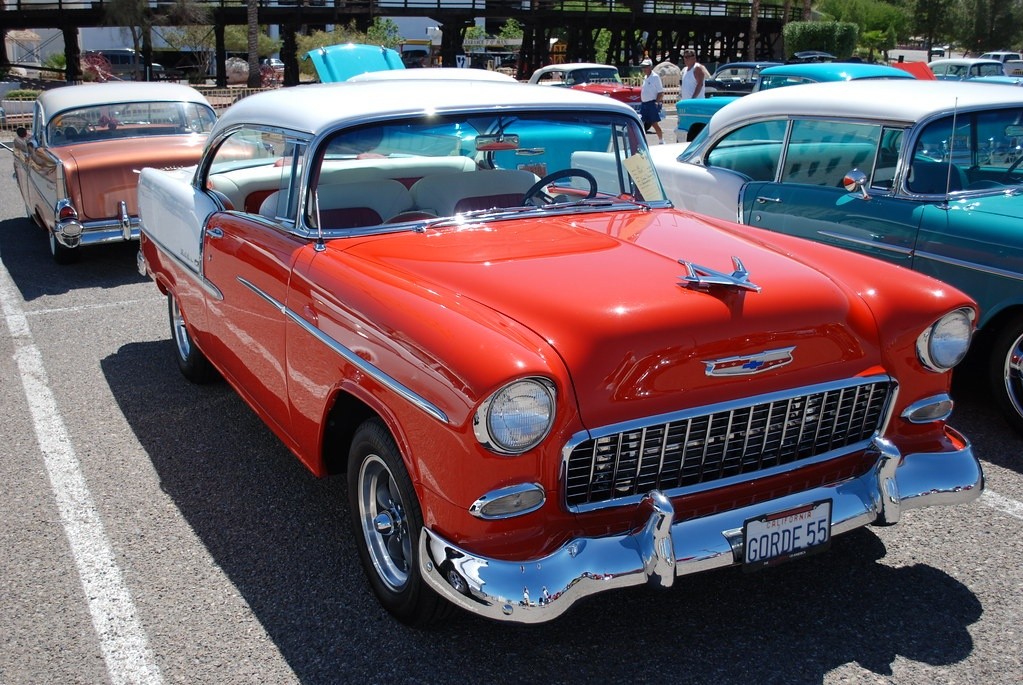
[411,168,550,217]
[259,180,415,229]
[907,163,968,193]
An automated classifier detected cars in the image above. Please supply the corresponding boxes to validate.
[15,79,261,266]
[132,73,986,630]
[564,78,1023,443]
[249,42,1022,202]
[263,58,284,82]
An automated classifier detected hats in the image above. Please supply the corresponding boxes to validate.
[640,58,652,65]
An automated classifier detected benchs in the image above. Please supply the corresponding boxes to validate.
[709,143,880,192]
[214,156,479,218]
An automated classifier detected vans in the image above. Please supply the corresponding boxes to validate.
[83,48,164,82]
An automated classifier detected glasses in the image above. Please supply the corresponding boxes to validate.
[684,54,693,58]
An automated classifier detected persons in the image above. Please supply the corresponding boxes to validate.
[680,49,706,100]
[639,58,665,145]
